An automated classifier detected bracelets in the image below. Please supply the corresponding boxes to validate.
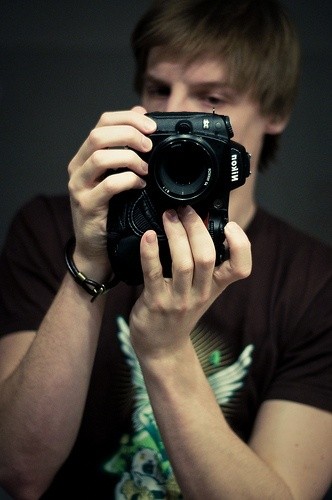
[61,235,122,305]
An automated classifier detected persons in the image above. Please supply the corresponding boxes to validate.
[1,0,331,499]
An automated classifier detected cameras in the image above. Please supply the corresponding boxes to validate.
[107,111,254,283]
